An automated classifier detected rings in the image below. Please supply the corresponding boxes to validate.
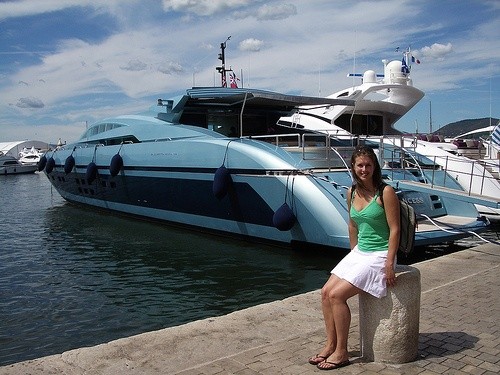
[394,278,396,280]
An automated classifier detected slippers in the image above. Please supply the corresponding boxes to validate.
[308,353,351,370]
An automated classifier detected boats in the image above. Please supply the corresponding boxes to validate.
[37,36,500,257]
[0,145,48,175]
[273,45,500,223]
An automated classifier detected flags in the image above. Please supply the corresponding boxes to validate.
[411,55,420,64]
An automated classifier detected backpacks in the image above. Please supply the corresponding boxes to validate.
[351,184,417,258]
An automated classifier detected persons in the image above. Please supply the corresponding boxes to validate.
[309,146,401,370]
